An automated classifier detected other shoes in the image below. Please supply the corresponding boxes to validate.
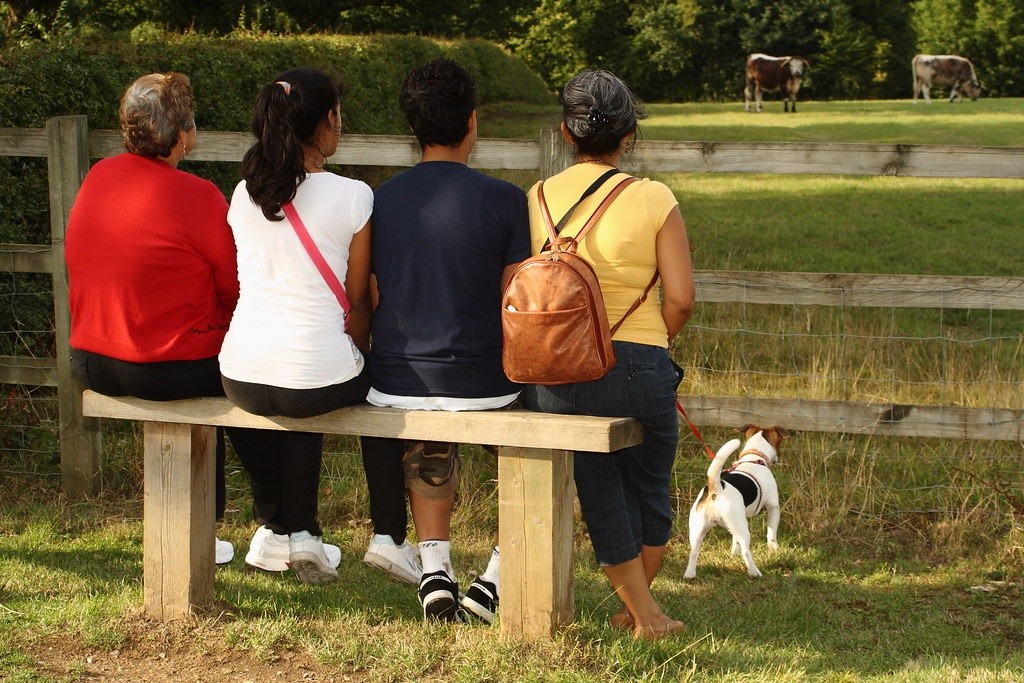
[611,614,687,640]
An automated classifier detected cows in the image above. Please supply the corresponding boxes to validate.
[911,53,987,106]
[743,53,811,113]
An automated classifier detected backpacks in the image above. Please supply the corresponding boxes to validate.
[500,175,660,383]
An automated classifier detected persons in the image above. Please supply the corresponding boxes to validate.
[368,58,532,626]
[216,68,373,586]
[527,69,695,639]
[64,74,243,565]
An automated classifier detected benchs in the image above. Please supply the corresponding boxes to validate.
[75,386,644,647]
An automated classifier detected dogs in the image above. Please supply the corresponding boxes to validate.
[682,424,794,580]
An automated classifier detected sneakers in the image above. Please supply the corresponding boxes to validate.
[418,570,458,624]
[287,530,339,585]
[363,534,424,589]
[244,525,341,573]
[215,536,234,564]
[460,575,500,628]
